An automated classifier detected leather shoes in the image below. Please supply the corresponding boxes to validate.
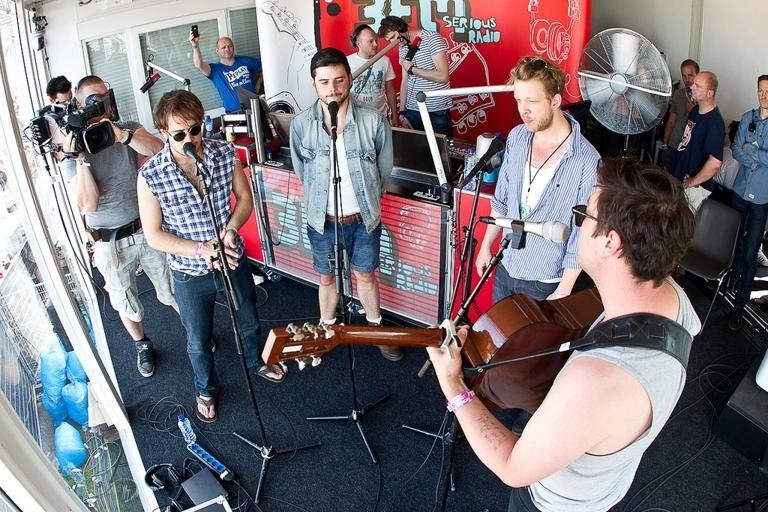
[298,317,343,366]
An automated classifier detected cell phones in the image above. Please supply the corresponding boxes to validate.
[192,25,198,37]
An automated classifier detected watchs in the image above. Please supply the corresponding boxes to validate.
[120,128,134,144]
[408,65,414,75]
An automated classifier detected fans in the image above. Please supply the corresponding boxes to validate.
[577,28,671,166]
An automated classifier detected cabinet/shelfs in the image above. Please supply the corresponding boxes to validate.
[213,121,504,328]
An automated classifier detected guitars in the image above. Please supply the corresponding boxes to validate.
[260,288,603,417]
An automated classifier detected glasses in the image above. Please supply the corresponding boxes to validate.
[166,123,201,142]
[570,204,597,227]
[520,56,547,71]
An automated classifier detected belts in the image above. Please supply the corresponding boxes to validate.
[96,218,141,270]
[326,215,360,223]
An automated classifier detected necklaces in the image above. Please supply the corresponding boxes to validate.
[526,128,571,191]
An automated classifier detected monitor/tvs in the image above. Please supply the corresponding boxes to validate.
[268,111,296,146]
[392,127,451,178]
[236,86,274,143]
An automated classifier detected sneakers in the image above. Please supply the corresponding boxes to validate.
[136,265,143,277]
[367,321,404,361]
[135,337,154,378]
[211,338,216,352]
[89,241,97,268]
[756,249,768,267]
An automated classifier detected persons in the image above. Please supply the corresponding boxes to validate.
[61,76,218,378]
[135,89,284,421]
[660,58,768,333]
[45,74,143,279]
[287,47,406,366]
[424,155,702,511]
[376,16,452,139]
[345,22,397,126]
[475,59,601,434]
[188,28,263,113]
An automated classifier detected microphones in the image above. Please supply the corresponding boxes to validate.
[404,36,422,61]
[458,138,506,188]
[327,100,339,142]
[140,73,160,93]
[182,142,212,179]
[479,216,569,244]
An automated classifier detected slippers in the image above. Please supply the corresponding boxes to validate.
[256,364,285,383]
[195,394,218,423]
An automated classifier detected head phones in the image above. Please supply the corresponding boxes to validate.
[108,479,139,503]
[144,462,182,491]
[349,24,367,48]
[161,498,186,512]
[380,19,408,32]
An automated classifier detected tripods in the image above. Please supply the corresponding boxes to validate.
[200,176,323,505]
[306,142,393,464]
[401,220,479,492]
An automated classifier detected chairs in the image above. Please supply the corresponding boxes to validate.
[677,197,741,336]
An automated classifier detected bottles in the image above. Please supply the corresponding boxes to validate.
[462,147,477,193]
[175,410,199,448]
[203,115,214,139]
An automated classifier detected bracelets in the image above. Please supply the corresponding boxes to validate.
[224,227,238,236]
[398,111,406,116]
[193,44,199,49]
[446,390,475,412]
[75,156,89,166]
[194,240,203,259]
